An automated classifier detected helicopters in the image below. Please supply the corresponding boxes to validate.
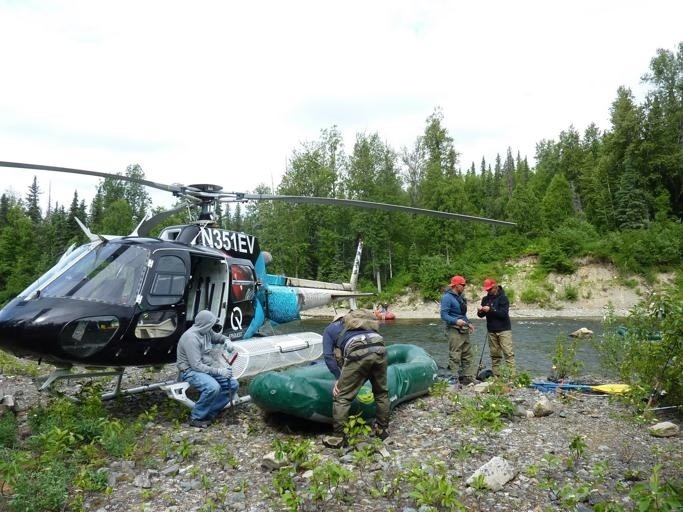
[0,161,518,413]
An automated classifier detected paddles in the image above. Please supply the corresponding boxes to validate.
[534,382,631,394]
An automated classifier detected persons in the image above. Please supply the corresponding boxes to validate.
[440,276,480,387]
[322,315,388,448]
[476,280,517,382]
[377,300,388,313]
[177,310,237,428]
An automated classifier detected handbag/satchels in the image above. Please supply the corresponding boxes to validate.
[448,325,469,334]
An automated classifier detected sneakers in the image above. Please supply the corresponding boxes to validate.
[375,428,390,439]
[322,433,347,449]
[188,418,210,428]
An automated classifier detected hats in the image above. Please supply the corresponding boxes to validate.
[333,313,343,322]
[447,275,464,286]
[481,279,497,291]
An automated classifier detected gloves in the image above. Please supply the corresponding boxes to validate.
[220,367,232,379]
[223,338,233,353]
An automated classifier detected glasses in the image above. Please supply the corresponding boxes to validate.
[459,282,465,286]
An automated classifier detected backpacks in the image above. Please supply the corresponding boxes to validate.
[344,309,380,332]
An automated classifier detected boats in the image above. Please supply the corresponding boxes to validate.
[251,343,437,426]
[619,327,662,340]
[375,304,395,319]
